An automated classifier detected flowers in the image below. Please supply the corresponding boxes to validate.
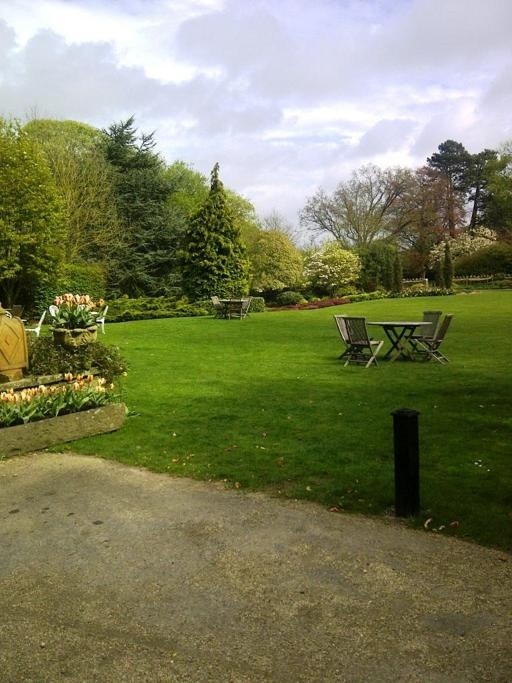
[48,293,106,329]
[0,371,115,426]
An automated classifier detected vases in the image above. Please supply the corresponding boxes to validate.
[47,325,101,349]
[0,400,128,458]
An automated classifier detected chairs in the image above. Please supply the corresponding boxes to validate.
[48,305,59,317]
[403,309,442,357]
[333,313,375,359]
[407,312,455,365]
[24,309,47,336]
[210,294,254,321]
[342,316,384,367]
[95,304,110,334]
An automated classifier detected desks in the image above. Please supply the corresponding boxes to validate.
[367,320,432,360]
